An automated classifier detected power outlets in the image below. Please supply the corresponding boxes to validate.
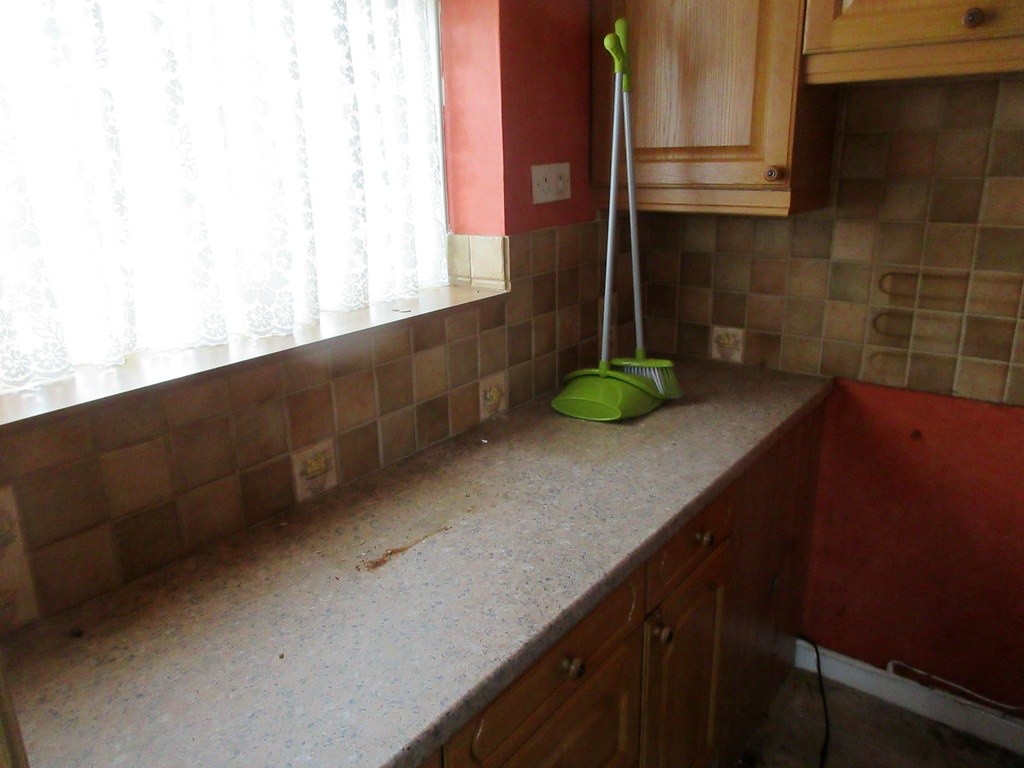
[530,162,570,204]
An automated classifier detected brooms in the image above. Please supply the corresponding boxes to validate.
[614,17,684,400]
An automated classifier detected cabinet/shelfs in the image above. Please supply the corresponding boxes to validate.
[591,0,831,218]
[419,409,826,768]
[802,0,1024,90]
